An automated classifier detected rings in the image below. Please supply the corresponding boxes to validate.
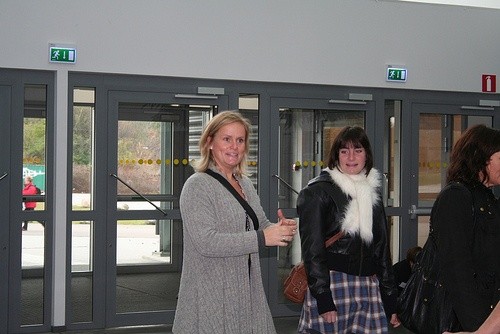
[282,235,286,241]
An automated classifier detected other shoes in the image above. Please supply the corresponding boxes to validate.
[22,226,27,231]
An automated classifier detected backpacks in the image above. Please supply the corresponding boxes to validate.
[35,187,41,195]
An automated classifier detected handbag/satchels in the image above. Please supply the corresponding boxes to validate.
[396,232,461,333]
[283,260,308,304]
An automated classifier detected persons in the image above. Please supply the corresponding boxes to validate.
[170,111,297,334]
[22,177,41,231]
[296,125,401,334]
[419,123,500,334]
